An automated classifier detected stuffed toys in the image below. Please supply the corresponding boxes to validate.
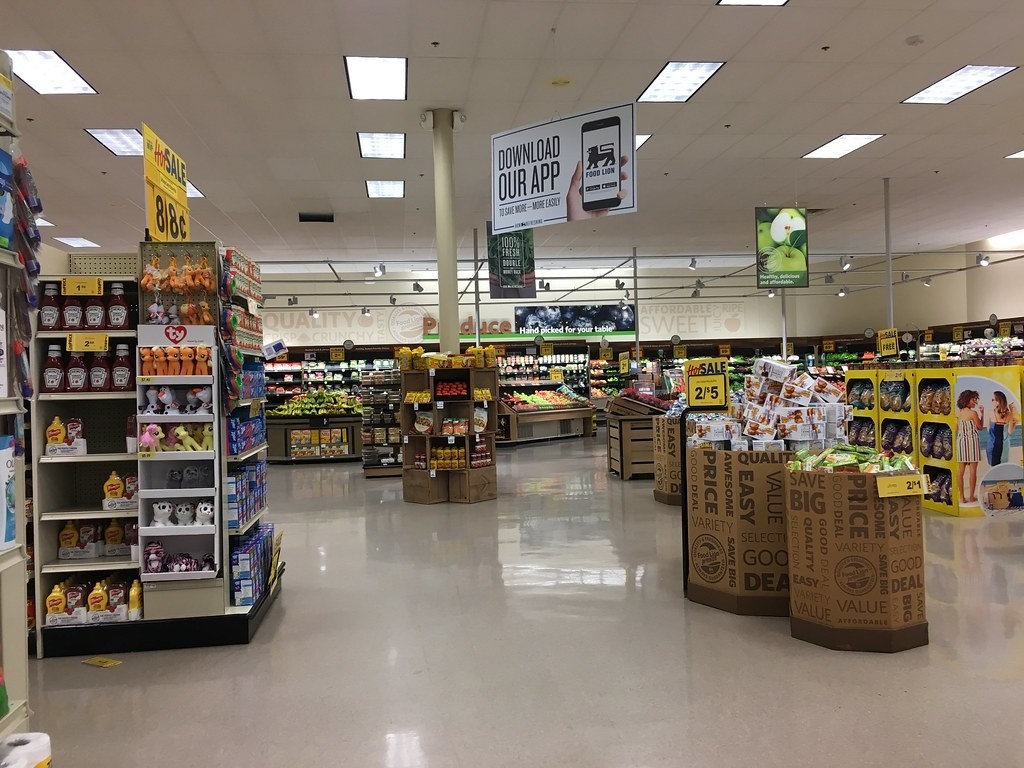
[140,255,214,573]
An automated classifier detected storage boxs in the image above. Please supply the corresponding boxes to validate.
[606,412,656,480]
[399,466,498,504]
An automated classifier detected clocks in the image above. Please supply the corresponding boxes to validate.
[864,328,875,338]
[989,313,997,326]
[671,335,680,345]
[534,336,544,346]
[343,340,354,350]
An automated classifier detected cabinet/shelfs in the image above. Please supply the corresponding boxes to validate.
[267,343,817,471]
[915,367,1022,516]
[845,369,879,451]
[876,368,918,465]
[0,278,281,754]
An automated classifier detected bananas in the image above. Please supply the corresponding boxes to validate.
[266,390,358,415]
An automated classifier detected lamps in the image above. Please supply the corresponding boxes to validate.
[623,290,630,300]
[308,307,319,319]
[835,289,845,297]
[373,263,386,277]
[362,307,371,316]
[287,296,298,305]
[619,301,628,310]
[618,282,625,290]
[389,295,397,304]
[688,258,697,270]
[921,278,932,287]
[976,254,990,266]
[742,289,775,298]
[839,257,850,271]
[413,282,424,292]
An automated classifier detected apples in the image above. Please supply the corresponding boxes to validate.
[771,208,806,245]
[768,246,806,271]
[758,223,776,251]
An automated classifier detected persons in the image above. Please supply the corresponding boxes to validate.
[957,389,984,503]
[986,391,1017,465]
[567,155,629,222]
